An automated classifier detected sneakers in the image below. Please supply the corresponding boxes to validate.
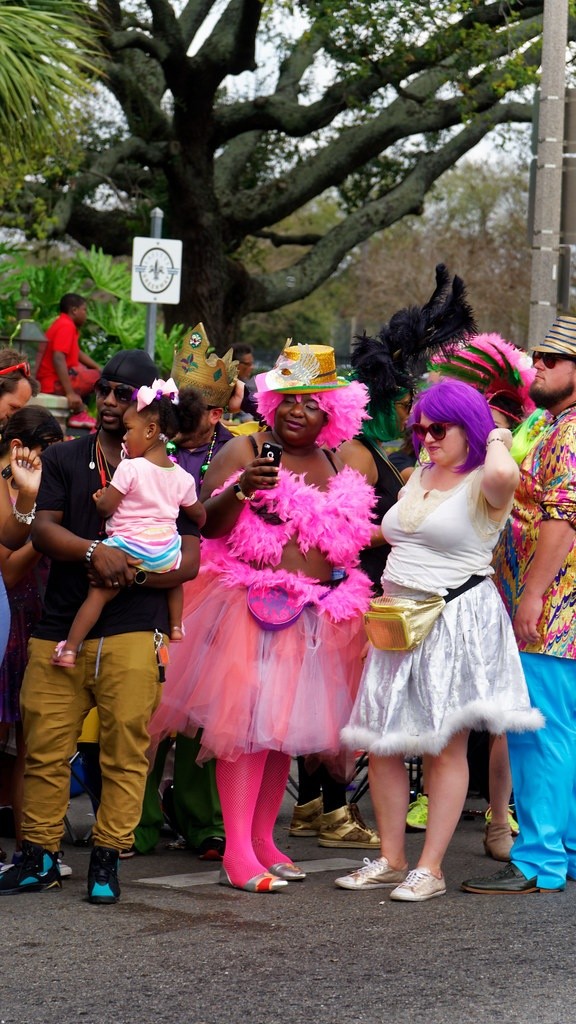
[390,868,448,902]
[87,847,121,903]
[283,794,323,836]
[316,802,382,849]
[0,841,64,896]
[405,793,429,829]
[335,856,411,890]
[484,806,519,836]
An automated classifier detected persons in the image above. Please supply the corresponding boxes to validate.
[0,292,576,905]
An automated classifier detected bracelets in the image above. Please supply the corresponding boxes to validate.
[85,539,102,563]
[486,437,506,451]
[12,500,36,525]
[234,479,256,503]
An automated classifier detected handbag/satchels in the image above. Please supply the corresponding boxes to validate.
[246,581,305,630]
[363,594,448,651]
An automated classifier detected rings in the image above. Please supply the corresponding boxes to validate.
[113,582,118,585]
[261,475,268,486]
[518,630,526,640]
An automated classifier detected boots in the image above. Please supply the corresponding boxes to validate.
[484,822,517,861]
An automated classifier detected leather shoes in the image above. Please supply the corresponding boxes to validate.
[460,863,564,894]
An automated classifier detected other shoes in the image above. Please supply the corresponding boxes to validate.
[119,844,137,858]
[195,836,224,861]
[219,857,307,892]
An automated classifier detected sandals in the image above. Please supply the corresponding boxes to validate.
[50,641,77,669]
[168,621,186,643]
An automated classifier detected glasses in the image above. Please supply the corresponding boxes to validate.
[394,399,412,411]
[532,350,576,370]
[0,360,32,377]
[410,421,457,441]
[95,378,133,404]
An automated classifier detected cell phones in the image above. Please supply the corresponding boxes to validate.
[259,442,282,486]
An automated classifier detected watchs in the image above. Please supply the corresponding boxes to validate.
[134,567,147,585]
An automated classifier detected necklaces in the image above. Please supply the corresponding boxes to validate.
[166,432,216,485]
[95,429,112,536]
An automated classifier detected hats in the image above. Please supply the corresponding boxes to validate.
[531,316,576,354]
[101,348,157,390]
[167,322,240,407]
[253,343,351,393]
[430,332,537,422]
